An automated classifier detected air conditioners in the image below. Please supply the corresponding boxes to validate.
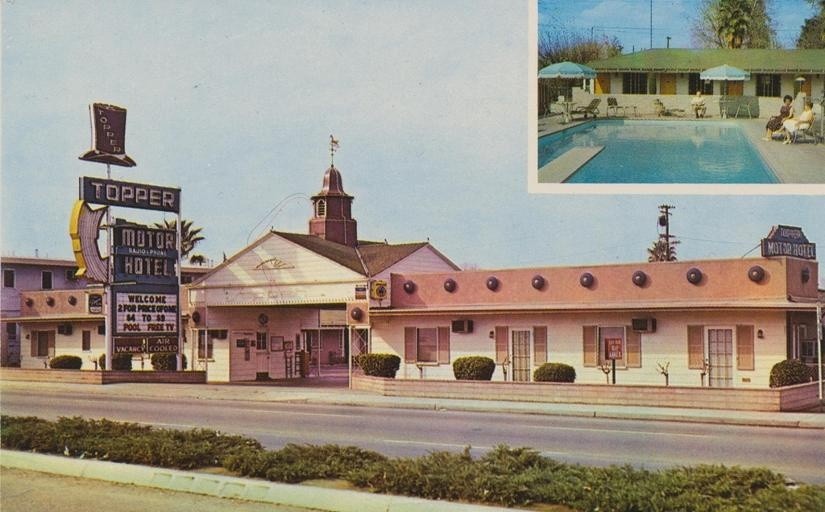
[631,316,656,333]
[451,319,473,331]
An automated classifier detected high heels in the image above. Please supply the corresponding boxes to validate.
[782,141,791,144]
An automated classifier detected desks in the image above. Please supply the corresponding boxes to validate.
[550,101,577,124]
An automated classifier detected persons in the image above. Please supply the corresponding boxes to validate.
[782,100,815,144]
[761,94,794,142]
[653,98,664,119]
[690,90,706,120]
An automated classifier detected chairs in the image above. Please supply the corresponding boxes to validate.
[774,113,820,145]
[570,97,753,120]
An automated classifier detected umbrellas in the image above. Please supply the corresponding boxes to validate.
[698,63,753,114]
[537,60,599,112]
